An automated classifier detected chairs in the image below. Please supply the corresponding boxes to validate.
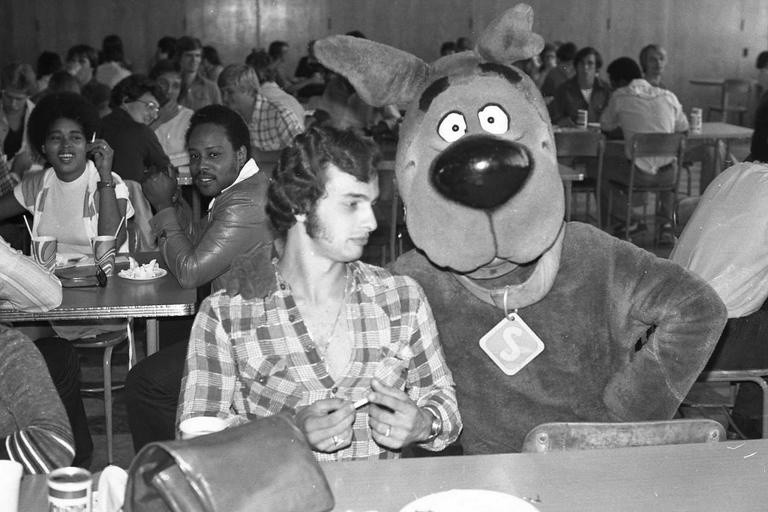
[69,180,156,464]
[521,418,726,452]
[671,197,767,439]
[606,130,684,245]
[707,79,753,126]
[552,129,605,230]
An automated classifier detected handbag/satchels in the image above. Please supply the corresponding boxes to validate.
[120,412,336,512]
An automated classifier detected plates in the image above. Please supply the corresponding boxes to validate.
[398,488,540,511]
[118,268,167,280]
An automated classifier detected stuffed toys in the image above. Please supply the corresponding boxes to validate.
[315,4,726,455]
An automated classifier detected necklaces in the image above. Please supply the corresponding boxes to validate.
[314,290,345,360]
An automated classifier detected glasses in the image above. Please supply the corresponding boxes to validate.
[576,60,595,66]
[543,55,556,59]
[135,99,162,117]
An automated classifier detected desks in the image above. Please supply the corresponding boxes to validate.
[689,77,751,123]
[175,171,202,224]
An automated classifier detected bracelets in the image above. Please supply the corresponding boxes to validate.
[103,145,107,150]
[96,181,116,189]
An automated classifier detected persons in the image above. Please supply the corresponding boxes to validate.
[518,37,578,100]
[32,35,131,99]
[218,62,305,151]
[439,37,475,58]
[150,60,197,164]
[751,50,767,124]
[1,323,77,478]
[0,91,136,268]
[295,40,315,80]
[0,234,65,313]
[126,105,276,459]
[154,35,177,66]
[596,56,689,246]
[173,36,222,112]
[245,52,305,125]
[636,43,669,90]
[173,123,464,465]
[269,41,323,93]
[0,62,38,181]
[204,47,227,83]
[548,47,609,136]
[94,72,172,190]
[658,125,767,444]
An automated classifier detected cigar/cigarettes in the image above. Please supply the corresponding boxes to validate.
[90,131,97,145]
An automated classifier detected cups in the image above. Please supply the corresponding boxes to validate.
[90,236,117,278]
[47,466,93,512]
[32,236,57,275]
[693,108,703,130]
[577,109,588,130]
[690,113,697,131]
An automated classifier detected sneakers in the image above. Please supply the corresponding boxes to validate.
[611,217,648,238]
[660,222,674,245]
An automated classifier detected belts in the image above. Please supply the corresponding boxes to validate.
[626,158,674,176]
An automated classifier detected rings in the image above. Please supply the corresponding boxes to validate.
[386,425,391,437]
[332,435,339,447]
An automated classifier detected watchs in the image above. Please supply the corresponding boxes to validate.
[422,406,441,440]
